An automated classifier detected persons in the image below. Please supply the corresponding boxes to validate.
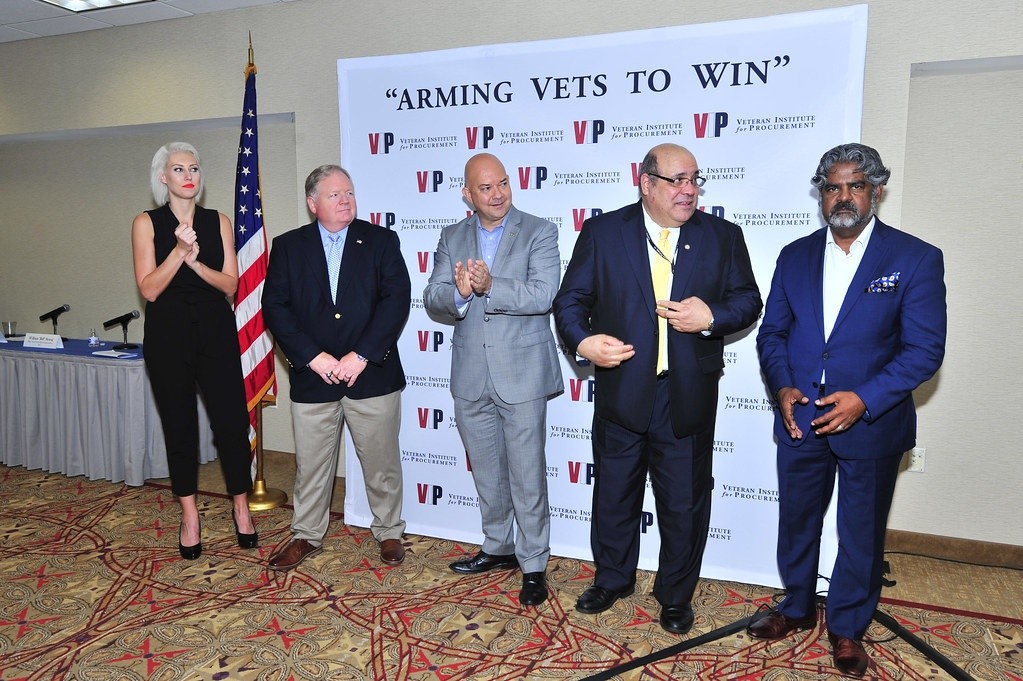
[260,165,411,573]
[553,143,765,635]
[747,139,947,681]
[133,142,258,560]
[423,152,565,605]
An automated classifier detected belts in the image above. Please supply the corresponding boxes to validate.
[656,370,669,382]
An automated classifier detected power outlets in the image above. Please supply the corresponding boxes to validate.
[907,448,926,472]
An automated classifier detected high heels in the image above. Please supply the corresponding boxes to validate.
[231,508,258,549]
[179,518,202,560]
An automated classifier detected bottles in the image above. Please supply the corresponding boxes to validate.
[87,328,99,347]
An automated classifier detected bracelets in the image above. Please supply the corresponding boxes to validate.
[708,318,715,331]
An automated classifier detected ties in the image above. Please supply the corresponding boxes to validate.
[327,234,340,306]
[650,229,670,375]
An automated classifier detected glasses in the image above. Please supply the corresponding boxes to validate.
[647,172,707,188]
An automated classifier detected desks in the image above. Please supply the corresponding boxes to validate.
[0,333,146,486]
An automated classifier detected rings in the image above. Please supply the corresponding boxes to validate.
[327,371,333,377]
[838,425,843,431]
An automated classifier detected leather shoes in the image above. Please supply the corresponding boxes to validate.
[518,570,548,606]
[660,602,694,634]
[448,549,520,574]
[746,610,817,641]
[828,629,868,678]
[380,539,406,565]
[576,580,636,614]
[267,539,323,571]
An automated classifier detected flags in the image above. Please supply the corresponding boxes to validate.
[229,75,277,491]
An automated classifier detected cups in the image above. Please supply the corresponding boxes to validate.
[2,322,16,337]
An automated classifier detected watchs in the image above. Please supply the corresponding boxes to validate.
[356,353,369,362]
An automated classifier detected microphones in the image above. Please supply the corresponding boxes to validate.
[39,303,71,321]
[103,310,140,327]
